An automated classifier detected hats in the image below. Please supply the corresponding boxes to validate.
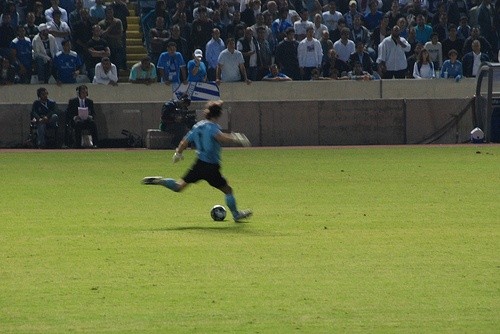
[194,49,203,57]
[349,0,357,6]
[38,23,50,31]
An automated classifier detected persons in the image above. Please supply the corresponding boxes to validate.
[32,87,67,149]
[440,50,462,79]
[461,40,489,76]
[216,39,251,84]
[262,64,291,81]
[160,94,194,148]
[0,0,130,84]
[64,84,98,148]
[92,57,118,86]
[143,0,500,81]
[413,49,435,79]
[377,25,411,79]
[157,43,188,85]
[187,49,207,81]
[206,28,225,81]
[142,101,252,221]
[129,56,158,84]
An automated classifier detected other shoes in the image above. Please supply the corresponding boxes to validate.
[233,209,253,222]
[141,176,162,185]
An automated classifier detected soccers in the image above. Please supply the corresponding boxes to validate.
[210,205,227,221]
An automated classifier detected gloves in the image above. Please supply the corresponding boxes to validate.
[232,134,251,146]
[173,148,184,164]
